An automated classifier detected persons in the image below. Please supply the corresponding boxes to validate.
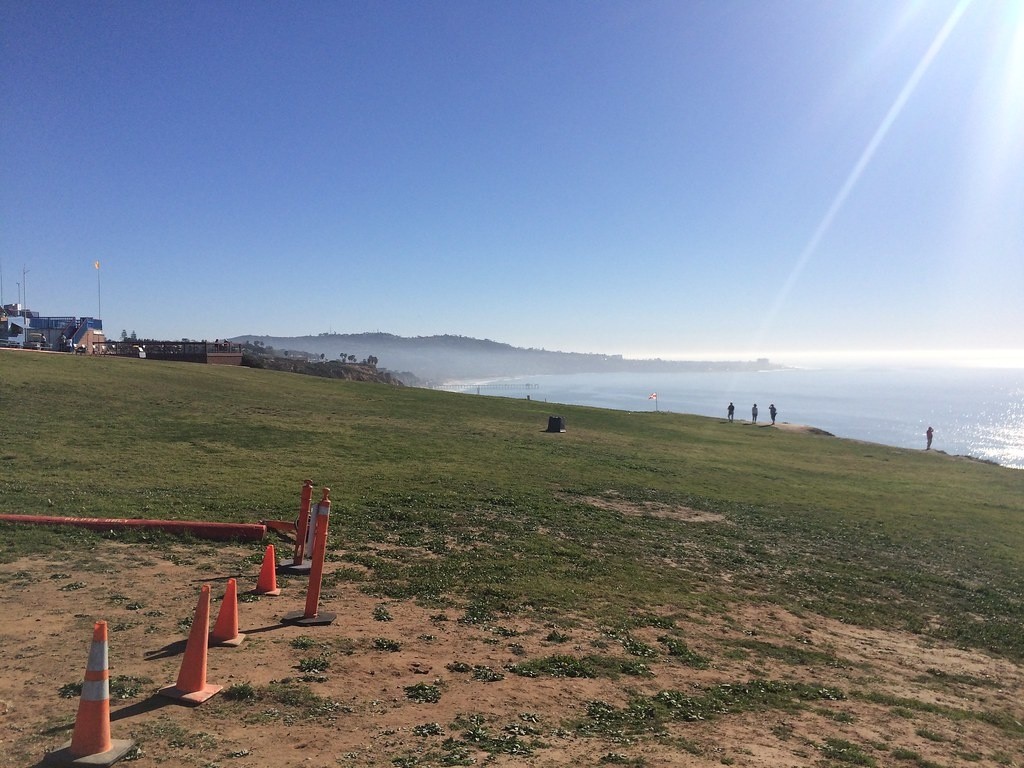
[769,404,777,426]
[751,404,758,424]
[727,402,734,423]
[926,427,934,450]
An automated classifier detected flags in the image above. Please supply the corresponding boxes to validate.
[648,393,656,399]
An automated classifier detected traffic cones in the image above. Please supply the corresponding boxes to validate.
[251,544,283,596]
[42,621,136,767]
[208,577,248,646]
[156,584,224,704]
